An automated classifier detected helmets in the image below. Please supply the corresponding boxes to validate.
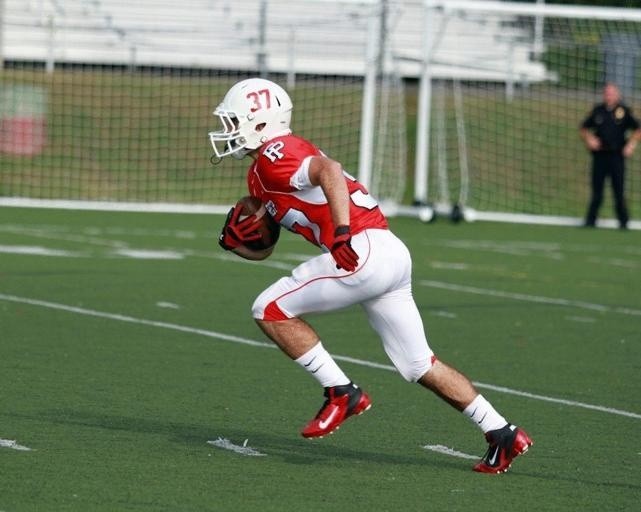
[207,78,294,166]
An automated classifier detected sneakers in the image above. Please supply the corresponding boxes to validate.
[302,380,373,439]
[472,423,534,475]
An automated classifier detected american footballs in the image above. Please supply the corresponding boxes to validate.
[237,196,280,250]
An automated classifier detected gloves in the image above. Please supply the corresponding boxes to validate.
[331,225,358,273]
[219,203,263,251]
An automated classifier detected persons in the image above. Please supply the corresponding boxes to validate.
[576,82,641,231]
[205,76,534,476]
